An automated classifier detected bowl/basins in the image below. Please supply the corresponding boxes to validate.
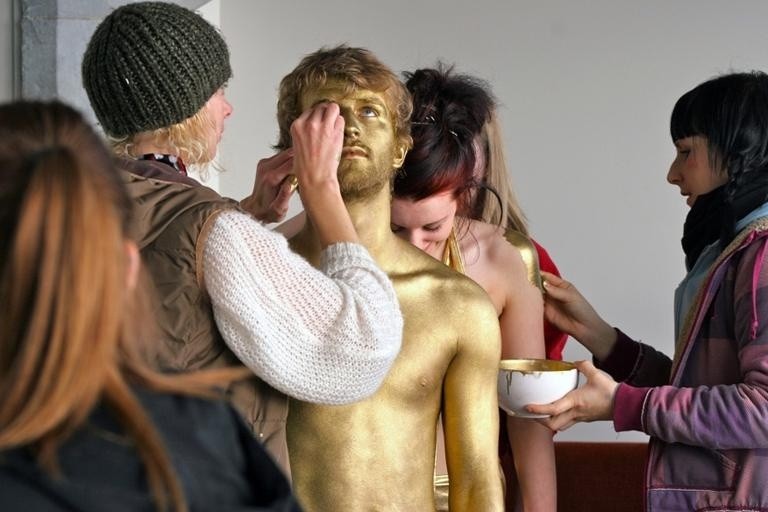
[497,358,579,421]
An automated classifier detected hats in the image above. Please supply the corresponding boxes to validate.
[81,2,231,134]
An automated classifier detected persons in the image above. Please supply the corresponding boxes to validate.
[402,105,570,512]
[269,43,506,512]
[524,70,768,512]
[272,60,558,512]
[0,99,304,512]
[81,1,404,486]
